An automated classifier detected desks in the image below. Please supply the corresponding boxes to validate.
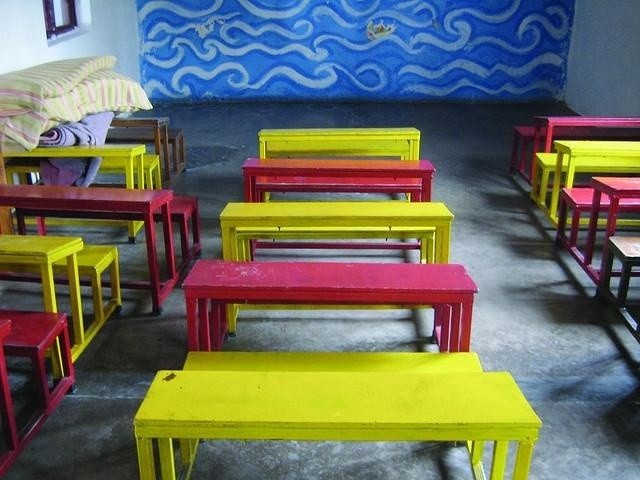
[133,369,544,480]
[110,117,177,189]
[0,184,186,316]
[0,319,46,480]
[0,234,101,394]
[523,116,640,188]
[0,144,145,243]
[182,258,478,352]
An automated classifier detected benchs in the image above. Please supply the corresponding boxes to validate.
[12,195,202,267]
[210,298,450,353]
[5,154,162,190]
[105,129,186,177]
[0,244,122,327]
[0,310,75,417]
[178,350,486,464]
[510,126,640,311]
